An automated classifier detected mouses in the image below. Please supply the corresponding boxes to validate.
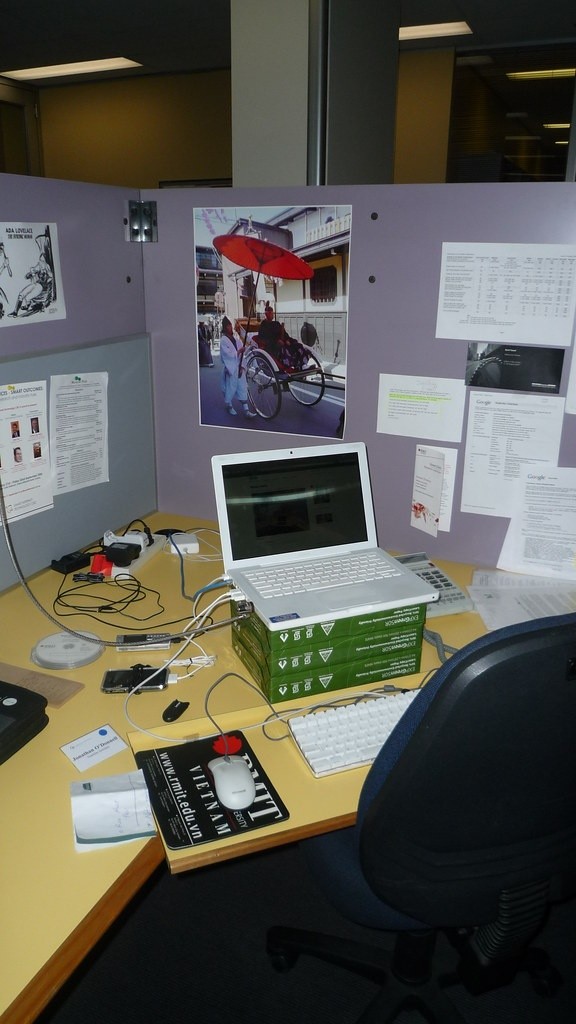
[208,754,256,811]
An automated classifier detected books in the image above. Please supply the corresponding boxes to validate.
[70,769,157,851]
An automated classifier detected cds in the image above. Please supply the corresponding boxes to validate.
[32,631,105,669]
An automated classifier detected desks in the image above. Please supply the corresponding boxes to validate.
[0,512,576,1024]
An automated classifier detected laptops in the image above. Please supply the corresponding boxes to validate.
[212,442,439,631]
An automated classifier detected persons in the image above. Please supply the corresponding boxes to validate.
[258,300,309,374]
[35,444,41,458]
[32,418,39,434]
[12,423,20,438]
[218,316,257,417]
[198,319,214,367]
[14,447,22,463]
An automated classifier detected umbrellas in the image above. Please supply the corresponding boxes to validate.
[213,233,314,373]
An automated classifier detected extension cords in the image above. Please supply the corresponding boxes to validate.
[111,533,167,579]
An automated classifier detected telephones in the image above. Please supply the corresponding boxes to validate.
[391,552,474,620]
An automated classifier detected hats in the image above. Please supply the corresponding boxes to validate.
[222,316,230,325]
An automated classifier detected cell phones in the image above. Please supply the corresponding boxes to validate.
[116,634,172,650]
[101,667,171,694]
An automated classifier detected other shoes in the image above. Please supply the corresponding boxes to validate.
[302,363,315,371]
[228,406,237,416]
[246,411,257,419]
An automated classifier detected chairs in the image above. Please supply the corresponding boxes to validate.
[264,609,576,1023]
[252,332,299,373]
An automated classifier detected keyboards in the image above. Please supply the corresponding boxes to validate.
[287,687,422,779]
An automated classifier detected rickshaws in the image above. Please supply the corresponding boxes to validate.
[234,316,345,420]
